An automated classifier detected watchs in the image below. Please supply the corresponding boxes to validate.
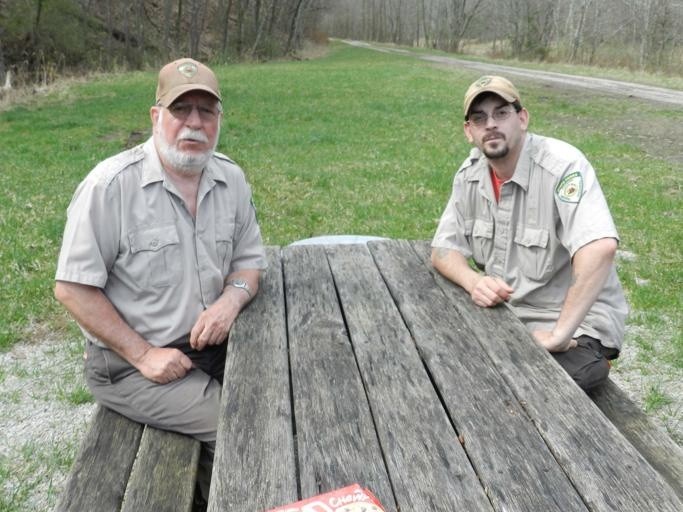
[223,278,254,300]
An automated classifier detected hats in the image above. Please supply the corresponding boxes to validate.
[462,75,519,118]
[153,57,222,109]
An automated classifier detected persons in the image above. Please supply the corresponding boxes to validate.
[53,59,268,504]
[430,74,629,394]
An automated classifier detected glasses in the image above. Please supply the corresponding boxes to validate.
[467,110,519,126]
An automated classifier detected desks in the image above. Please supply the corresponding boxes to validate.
[207,239,681,512]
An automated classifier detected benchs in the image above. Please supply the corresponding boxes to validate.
[586,375,681,502]
[55,405,202,512]
